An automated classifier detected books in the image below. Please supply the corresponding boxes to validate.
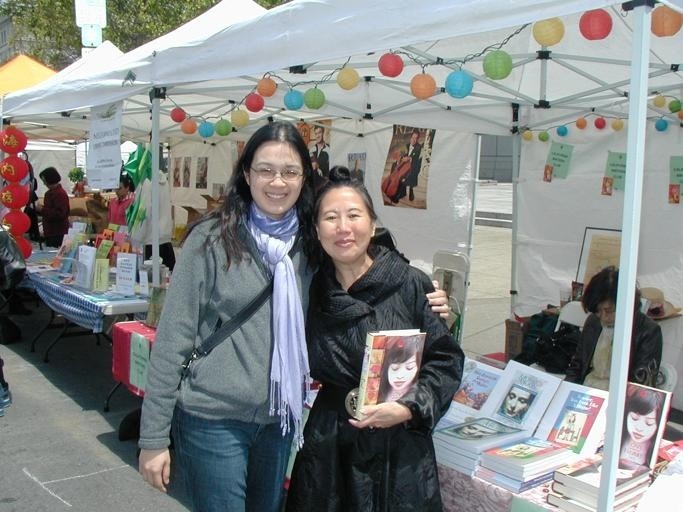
[357,328,426,418]
[432,359,683,511]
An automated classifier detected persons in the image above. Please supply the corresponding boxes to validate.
[564,266,663,390]
[308,122,329,177]
[108,171,134,225]
[133,171,176,270]
[284,165,465,510]
[350,159,363,182]
[35,166,70,247]
[371,334,424,401]
[391,129,421,202]
[0,230,26,418]
[137,120,455,511]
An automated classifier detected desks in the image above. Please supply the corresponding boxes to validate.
[24,241,170,413]
[437,445,654,512]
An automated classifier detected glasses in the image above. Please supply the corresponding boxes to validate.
[247,165,305,181]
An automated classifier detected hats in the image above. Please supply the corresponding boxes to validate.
[638,287,674,320]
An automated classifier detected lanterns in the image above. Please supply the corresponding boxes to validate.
[11,235,32,260]
[0,125,27,155]
[0,182,29,208]
[2,208,31,235]
[0,155,28,182]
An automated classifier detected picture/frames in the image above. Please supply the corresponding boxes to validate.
[576,226,621,297]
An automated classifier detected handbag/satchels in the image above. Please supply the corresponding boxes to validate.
[0,230,26,290]
[525,328,581,372]
